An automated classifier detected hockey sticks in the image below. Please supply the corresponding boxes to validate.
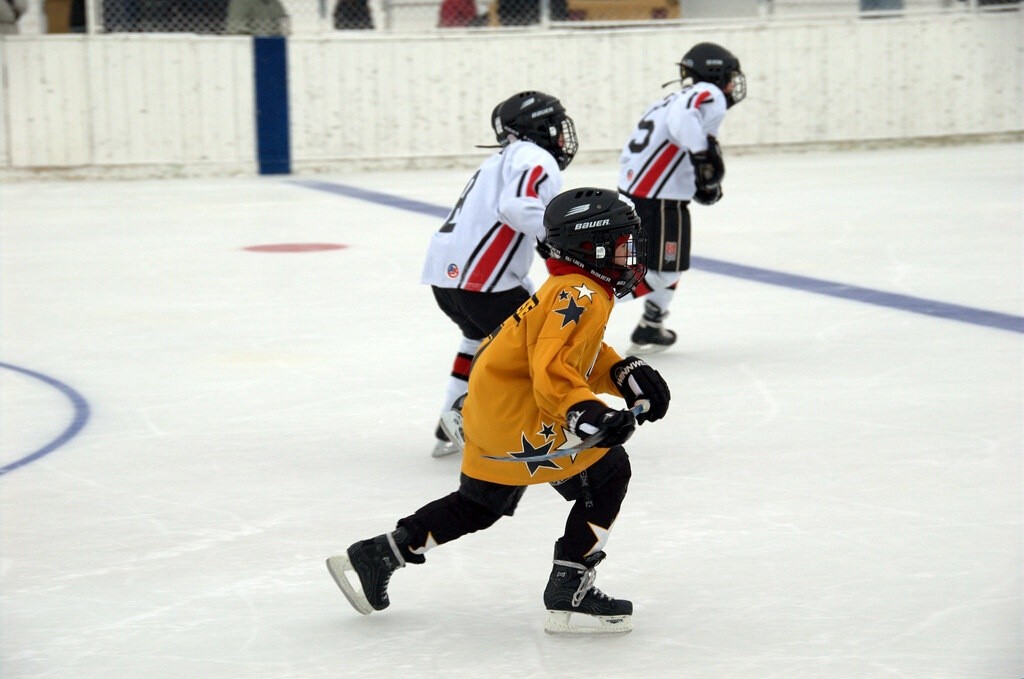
[480,398,651,463]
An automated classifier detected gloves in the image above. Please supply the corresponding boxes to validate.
[689,134,725,186]
[565,400,635,448]
[693,182,723,206]
[610,355,670,425]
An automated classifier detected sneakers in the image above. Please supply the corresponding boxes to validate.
[543,540,632,634]
[625,299,677,357]
[325,526,426,615]
[433,393,468,457]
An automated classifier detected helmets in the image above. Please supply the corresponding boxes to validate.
[491,90,579,171]
[543,188,647,299]
[680,42,746,109]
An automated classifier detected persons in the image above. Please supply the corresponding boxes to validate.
[346,185,671,617]
[421,90,579,443]
[618,41,745,347]
